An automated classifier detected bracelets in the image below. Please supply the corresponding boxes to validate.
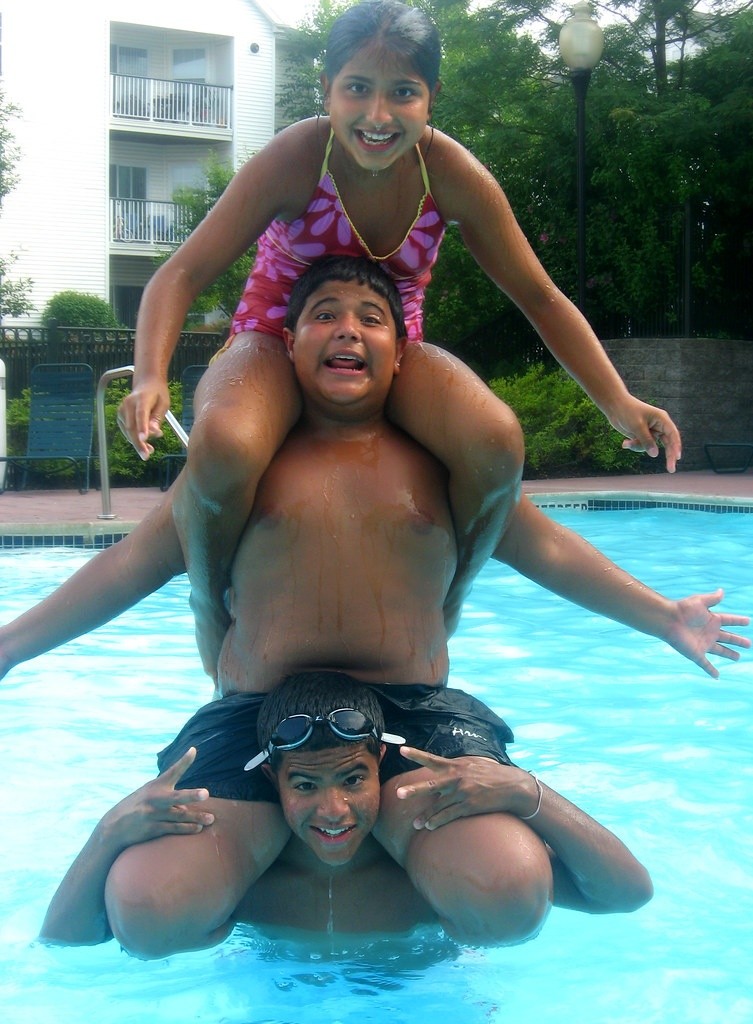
[520,770,543,820]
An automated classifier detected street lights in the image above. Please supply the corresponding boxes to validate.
[553,2,606,319]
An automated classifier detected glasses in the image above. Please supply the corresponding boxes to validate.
[266,707,383,753]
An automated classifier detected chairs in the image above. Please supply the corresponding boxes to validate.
[0,363,98,493]
[124,213,177,242]
[158,365,208,490]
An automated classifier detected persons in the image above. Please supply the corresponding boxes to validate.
[0,253,751,952]
[40,670,653,944]
[118,0,682,683]
